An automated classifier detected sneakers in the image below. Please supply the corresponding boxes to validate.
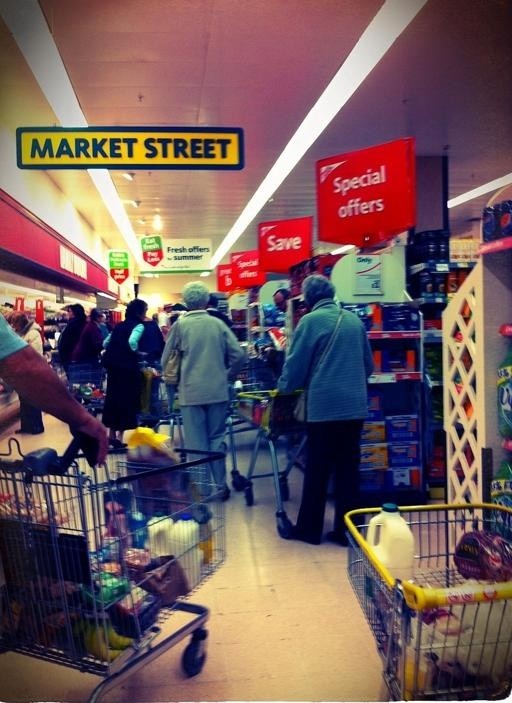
[281,525,322,545]
[326,530,361,547]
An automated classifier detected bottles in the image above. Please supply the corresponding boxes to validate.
[412,226,470,304]
[147,501,213,585]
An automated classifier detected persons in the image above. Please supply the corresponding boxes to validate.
[0,282,244,502]
[278,274,373,547]
[273,289,292,313]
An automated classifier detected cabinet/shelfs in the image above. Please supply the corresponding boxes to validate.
[219,255,303,423]
[480,184,511,550]
[356,260,478,504]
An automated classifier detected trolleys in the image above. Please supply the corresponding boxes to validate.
[68,360,108,418]
[144,362,312,539]
[340,498,511,701]
[0,433,238,700]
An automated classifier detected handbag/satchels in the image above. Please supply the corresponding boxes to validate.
[293,391,306,422]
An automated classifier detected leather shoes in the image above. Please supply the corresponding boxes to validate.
[108,438,128,448]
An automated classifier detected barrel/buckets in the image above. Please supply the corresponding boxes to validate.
[147,511,172,556]
[172,513,200,590]
[367,503,414,584]
